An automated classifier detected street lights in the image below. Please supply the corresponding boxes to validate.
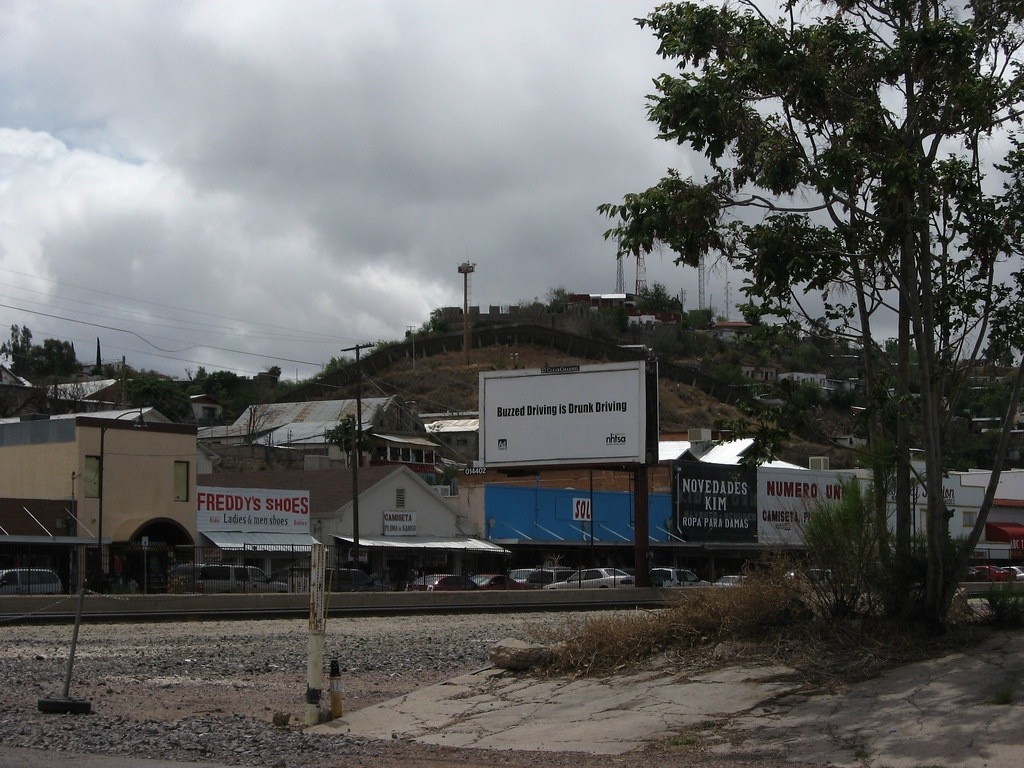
[96,405,152,577]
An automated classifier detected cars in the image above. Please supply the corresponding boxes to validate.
[543,566,636,590]
[999,566,1024,581]
[471,574,526,590]
[974,564,1012,582]
[964,567,988,583]
[713,574,748,588]
[405,573,478,591]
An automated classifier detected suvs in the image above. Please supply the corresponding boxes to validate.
[651,566,713,587]
[0,568,64,594]
[326,566,393,593]
[511,567,578,590]
[170,559,290,592]
